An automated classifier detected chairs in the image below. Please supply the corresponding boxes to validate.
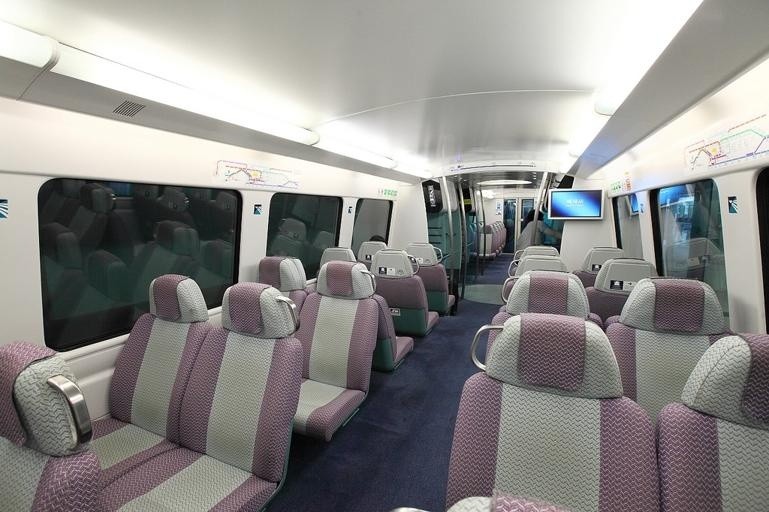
[97,280,305,510]
[295,261,381,449]
[659,332,768,512]
[447,308,664,510]
[3,341,97,510]
[256,216,726,409]
[91,271,213,489]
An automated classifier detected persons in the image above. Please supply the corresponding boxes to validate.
[369,235,386,243]
[517,209,563,262]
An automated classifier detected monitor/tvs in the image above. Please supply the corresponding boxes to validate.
[548,189,605,220]
[624,184,694,216]
